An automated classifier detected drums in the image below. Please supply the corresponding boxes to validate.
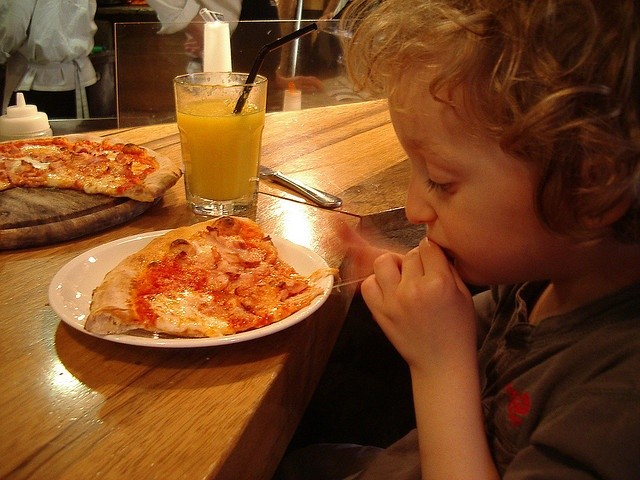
[259,161,344,208]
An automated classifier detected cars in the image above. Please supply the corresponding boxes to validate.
[174,75,268,217]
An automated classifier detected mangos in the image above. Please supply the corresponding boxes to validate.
[84,213,326,337]
[2,135,182,225]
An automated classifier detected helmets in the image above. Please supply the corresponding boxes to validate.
[46,231,332,350]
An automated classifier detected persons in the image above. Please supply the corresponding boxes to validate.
[0,0,101,119]
[147,0,283,84]
[277,0,637,480]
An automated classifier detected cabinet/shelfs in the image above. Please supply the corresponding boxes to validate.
[0,92,52,145]
